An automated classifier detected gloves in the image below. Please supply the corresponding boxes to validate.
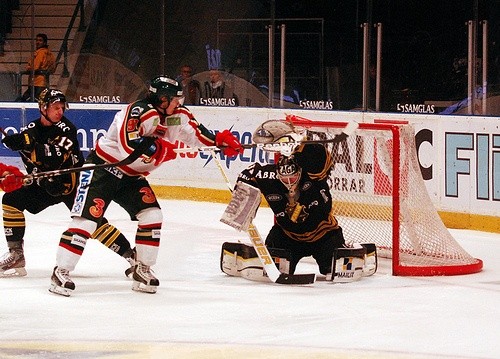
[153,140,178,165]
[41,177,65,196]
[0,163,24,193]
[215,130,242,156]
[2,133,34,153]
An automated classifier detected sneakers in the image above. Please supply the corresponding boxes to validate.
[125,247,136,278]
[0,242,27,277]
[48,265,75,297]
[131,262,159,294]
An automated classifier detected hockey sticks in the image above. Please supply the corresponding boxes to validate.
[173,117,359,154]
[209,149,317,285]
[0,127,68,192]
[0,136,159,181]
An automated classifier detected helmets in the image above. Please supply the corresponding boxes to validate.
[149,76,183,104]
[276,156,302,189]
[39,88,66,109]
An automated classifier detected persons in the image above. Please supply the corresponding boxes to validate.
[25,33,56,102]
[203,70,226,105]
[179,65,204,107]
[0,87,137,278]
[49,76,244,298]
[219,121,378,283]
